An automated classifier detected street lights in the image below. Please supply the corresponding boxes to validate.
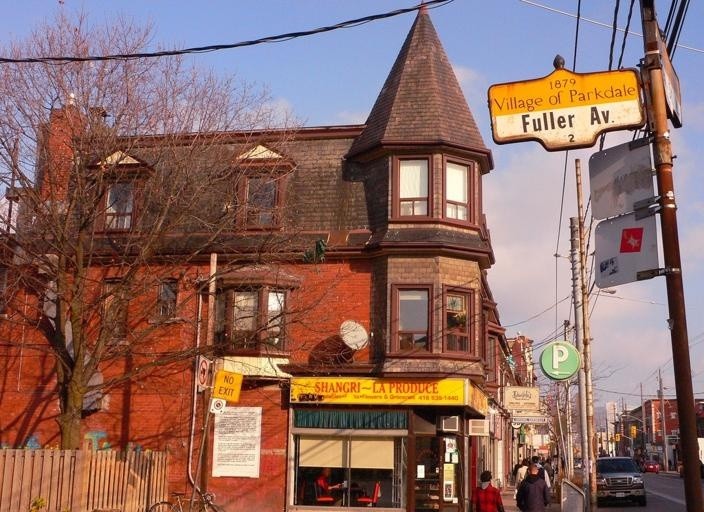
[620,435,633,457]
[621,414,647,459]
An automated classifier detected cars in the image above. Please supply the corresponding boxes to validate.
[641,460,660,475]
[574,461,582,468]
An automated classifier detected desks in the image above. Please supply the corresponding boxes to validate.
[331,486,365,507]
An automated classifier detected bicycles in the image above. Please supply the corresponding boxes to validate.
[149,486,227,512]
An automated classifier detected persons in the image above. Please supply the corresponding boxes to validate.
[513,455,557,512]
[315,467,343,507]
[470,471,504,512]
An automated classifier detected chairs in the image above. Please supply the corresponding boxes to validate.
[358,481,382,506]
[314,482,335,506]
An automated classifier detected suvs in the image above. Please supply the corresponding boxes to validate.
[595,457,648,507]
